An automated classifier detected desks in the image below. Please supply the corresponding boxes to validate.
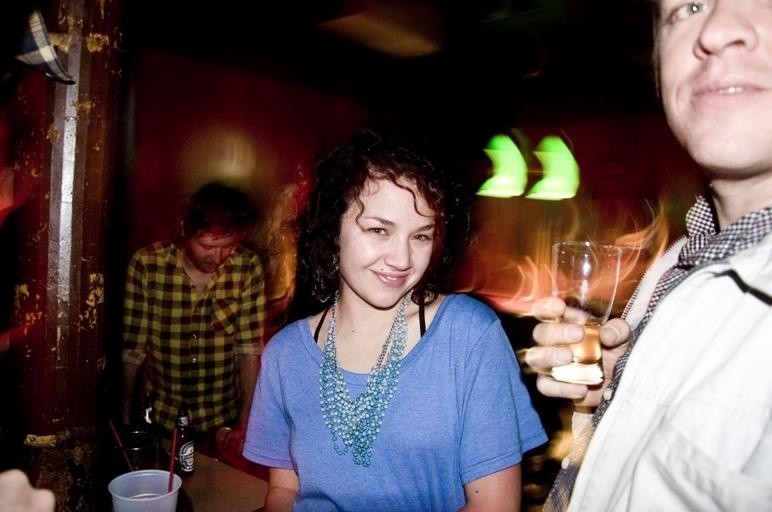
[177,451,268,512]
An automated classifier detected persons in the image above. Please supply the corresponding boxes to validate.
[120,178,266,473]
[524,0,772,512]
[241,129,550,511]
[0,468,56,512]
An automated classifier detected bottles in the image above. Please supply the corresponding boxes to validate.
[176,404,196,478]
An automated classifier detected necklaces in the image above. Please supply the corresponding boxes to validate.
[319,282,418,469]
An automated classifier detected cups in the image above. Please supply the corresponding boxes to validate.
[550,242,624,387]
[108,431,161,468]
[108,469,182,512]
